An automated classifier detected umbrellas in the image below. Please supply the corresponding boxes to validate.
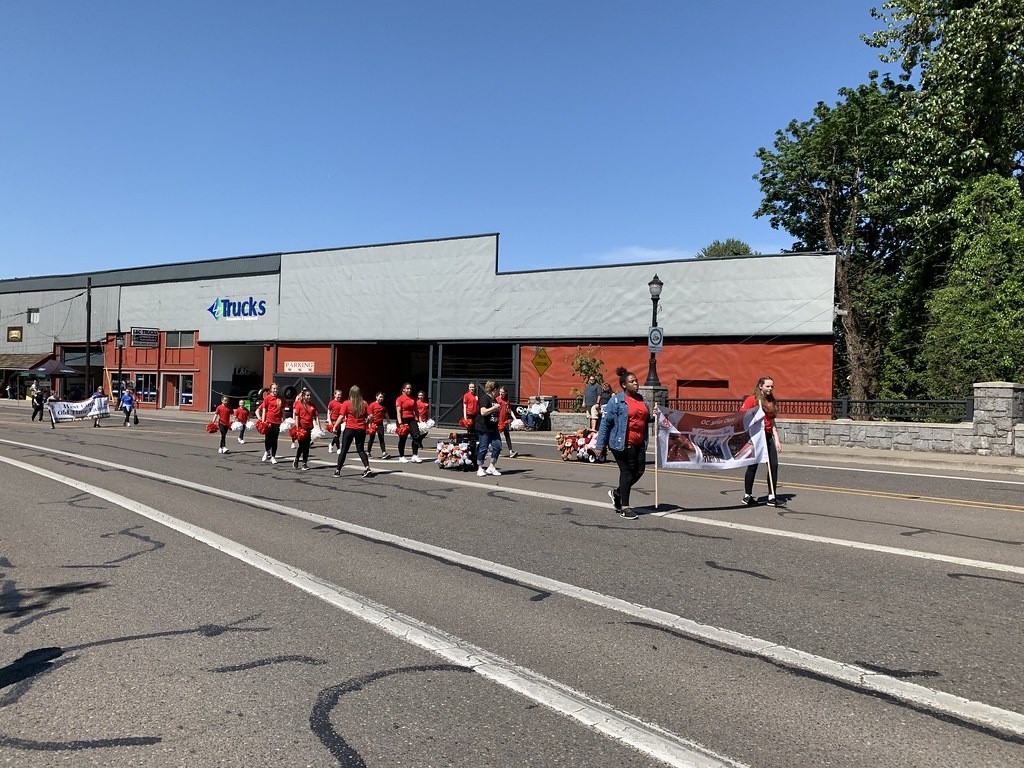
[17,359,82,391]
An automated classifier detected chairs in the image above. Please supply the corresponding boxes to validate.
[528,401,550,430]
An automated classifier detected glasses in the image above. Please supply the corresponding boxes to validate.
[589,379,595,381]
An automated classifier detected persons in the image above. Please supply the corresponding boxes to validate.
[211,395,251,454]
[92,385,108,428]
[521,395,547,432]
[594,366,658,520]
[582,374,616,431]
[255,382,288,465]
[291,386,322,471]
[29,379,60,429]
[740,376,787,507]
[118,389,135,428]
[326,385,391,478]
[463,381,518,458]
[395,383,428,464]
[474,380,502,477]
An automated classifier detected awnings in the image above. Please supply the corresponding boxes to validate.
[0,353,54,371]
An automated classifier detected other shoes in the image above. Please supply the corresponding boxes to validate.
[238,435,245,444]
[123,422,131,428]
[382,453,390,459]
[218,447,229,454]
[361,468,372,478]
[261,450,277,465]
[310,441,314,448]
[293,457,310,472]
[526,427,534,430]
[290,443,296,449]
[328,443,341,455]
[367,453,373,458]
[333,470,340,478]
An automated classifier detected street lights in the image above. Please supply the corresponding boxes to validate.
[643,272,664,386]
[114,335,124,411]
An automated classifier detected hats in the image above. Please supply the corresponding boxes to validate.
[535,396,541,400]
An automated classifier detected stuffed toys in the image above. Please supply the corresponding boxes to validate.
[555,428,608,460]
[434,432,487,468]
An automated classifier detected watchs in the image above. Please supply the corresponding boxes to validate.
[596,402,600,405]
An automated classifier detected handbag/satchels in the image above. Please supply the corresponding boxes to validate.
[134,409,139,425]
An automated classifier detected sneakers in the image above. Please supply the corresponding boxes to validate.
[499,452,505,457]
[509,450,518,457]
[477,467,501,477]
[619,507,638,520]
[412,455,422,463]
[766,497,786,506]
[398,456,409,463]
[742,494,759,506]
[608,489,621,511]
